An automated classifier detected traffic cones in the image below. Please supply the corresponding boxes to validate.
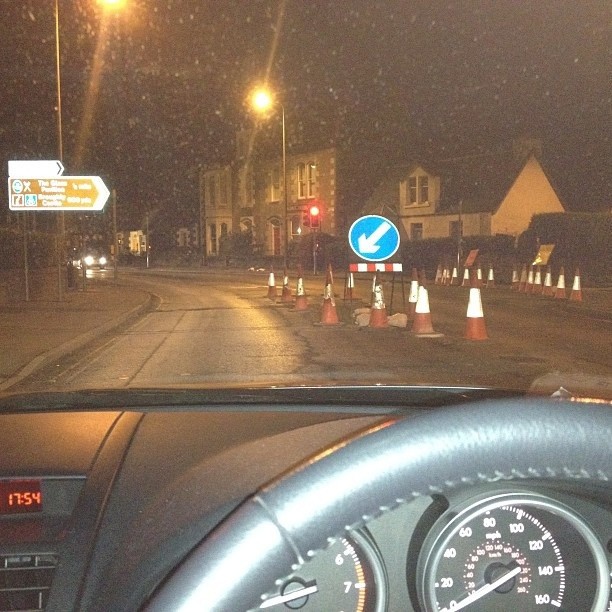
[282,266,291,302]
[450,266,458,286]
[440,268,449,286]
[487,263,495,289]
[369,282,388,329]
[325,263,334,289]
[511,263,519,293]
[476,264,483,288]
[265,265,277,299]
[526,265,534,295]
[569,268,582,302]
[296,277,306,311]
[555,266,566,299]
[542,265,553,296]
[462,266,471,287]
[533,264,542,295]
[519,264,527,293]
[343,273,356,302]
[322,284,337,324]
[465,270,488,341]
[411,285,433,335]
[408,268,419,315]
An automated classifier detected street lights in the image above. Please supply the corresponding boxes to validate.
[306,203,321,277]
[54,0,125,228]
[247,85,293,300]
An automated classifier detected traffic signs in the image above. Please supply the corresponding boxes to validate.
[6,155,112,214]
